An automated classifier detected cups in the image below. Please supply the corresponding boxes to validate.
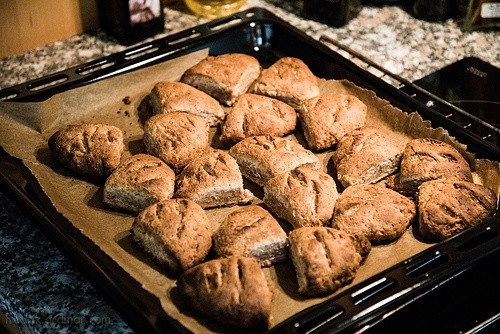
[94,0,165,47]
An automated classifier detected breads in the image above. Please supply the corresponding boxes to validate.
[219,93,297,148]
[226,134,319,186]
[213,205,289,268]
[331,184,417,242]
[103,153,176,214]
[298,93,369,151]
[152,80,226,126]
[400,138,473,197]
[262,161,341,229]
[48,122,124,176]
[180,53,262,107]
[130,198,212,272]
[332,124,400,188]
[175,254,272,334]
[248,57,320,111]
[287,226,371,296]
[144,111,210,173]
[417,179,499,242]
[174,149,253,209]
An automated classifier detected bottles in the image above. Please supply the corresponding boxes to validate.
[184,0,247,19]
